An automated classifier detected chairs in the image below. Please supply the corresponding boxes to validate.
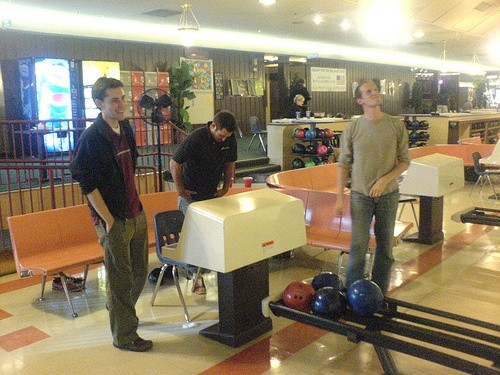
[245,115,267,152]
[470,151,499,201]
[149,209,197,323]
[398,196,420,230]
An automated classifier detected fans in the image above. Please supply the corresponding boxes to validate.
[137,88,175,192]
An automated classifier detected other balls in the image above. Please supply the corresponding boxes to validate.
[291,127,334,139]
[291,142,334,155]
[408,131,430,140]
[291,158,332,170]
[282,271,384,322]
[401,119,429,128]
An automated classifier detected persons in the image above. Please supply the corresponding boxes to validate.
[289,78,312,113]
[285,94,319,117]
[69,76,153,351]
[170,109,238,294]
[333,77,411,296]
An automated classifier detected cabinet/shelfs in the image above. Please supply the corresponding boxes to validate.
[399,113,432,147]
[291,120,335,168]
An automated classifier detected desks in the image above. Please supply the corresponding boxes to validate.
[10,127,84,182]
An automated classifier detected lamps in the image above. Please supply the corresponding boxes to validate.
[175,3,200,48]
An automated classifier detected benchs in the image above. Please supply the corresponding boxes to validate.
[6,142,498,319]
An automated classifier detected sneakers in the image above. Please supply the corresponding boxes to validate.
[52,273,83,292]
[193,277,206,293]
[113,337,153,352]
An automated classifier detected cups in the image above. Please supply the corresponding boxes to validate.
[243,177,253,187]
[295,112,302,120]
[306,111,312,119]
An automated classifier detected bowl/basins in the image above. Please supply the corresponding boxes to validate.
[313,113,325,118]
